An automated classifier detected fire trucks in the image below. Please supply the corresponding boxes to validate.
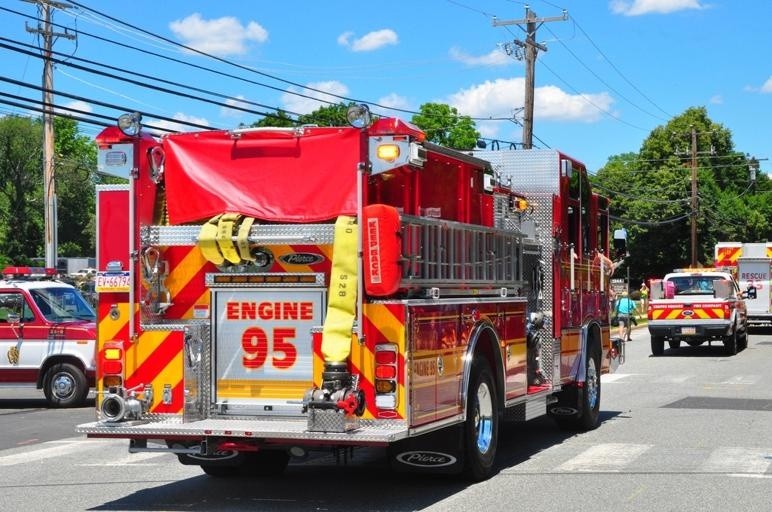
[75,105,617,484]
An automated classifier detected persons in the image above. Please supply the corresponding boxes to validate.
[640,285,650,312]
[741,279,756,299]
[608,285,627,311]
[598,253,616,276]
[614,290,642,341]
[639,283,649,292]
[736,289,744,299]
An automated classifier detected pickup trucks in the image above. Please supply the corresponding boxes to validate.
[647,268,756,356]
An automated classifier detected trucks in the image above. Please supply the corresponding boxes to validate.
[714,241,772,332]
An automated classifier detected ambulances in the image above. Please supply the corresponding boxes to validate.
[0,265,97,408]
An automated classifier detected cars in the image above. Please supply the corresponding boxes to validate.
[50,267,98,280]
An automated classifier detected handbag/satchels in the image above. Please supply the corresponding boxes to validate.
[613,317,618,326]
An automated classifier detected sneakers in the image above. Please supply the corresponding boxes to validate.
[627,339,632,341]
